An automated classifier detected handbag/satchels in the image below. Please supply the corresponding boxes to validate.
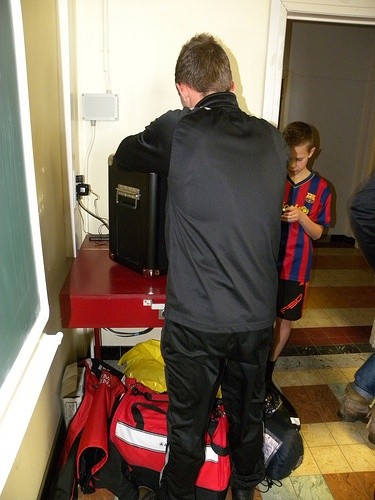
[109,378,231,493]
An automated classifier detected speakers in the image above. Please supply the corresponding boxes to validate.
[108,155,169,277]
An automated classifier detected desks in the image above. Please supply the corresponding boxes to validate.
[59,232,167,358]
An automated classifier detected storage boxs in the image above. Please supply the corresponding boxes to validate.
[61,362,86,432]
[108,153,167,279]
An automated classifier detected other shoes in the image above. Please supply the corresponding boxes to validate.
[337,381,370,424]
[366,403,375,444]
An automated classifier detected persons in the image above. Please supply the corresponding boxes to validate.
[339,167,375,446]
[265,122,331,392]
[113,36,291,500]
